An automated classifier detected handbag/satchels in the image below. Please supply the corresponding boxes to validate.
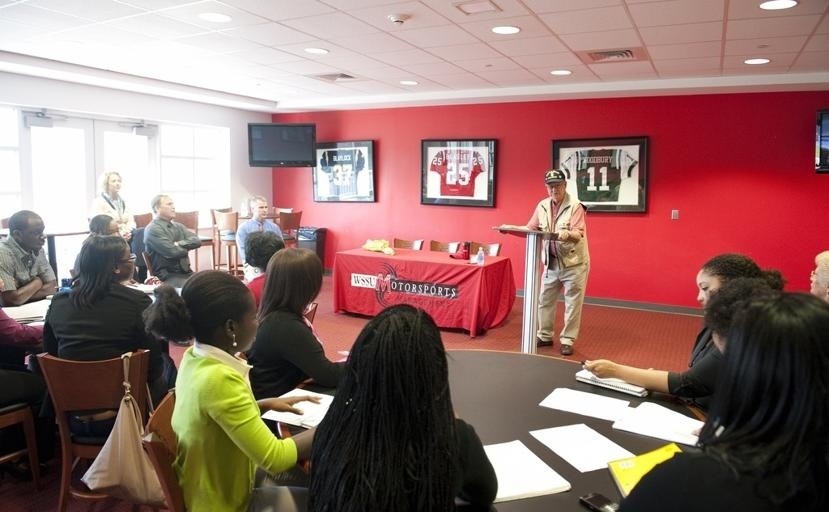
[80,393,168,507]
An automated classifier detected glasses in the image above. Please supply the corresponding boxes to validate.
[120,254,137,263]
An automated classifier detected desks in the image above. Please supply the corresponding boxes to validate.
[278,350,719,512]
[332,247,516,338]
[1,283,186,329]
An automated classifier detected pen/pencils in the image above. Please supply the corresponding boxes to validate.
[580,360,588,366]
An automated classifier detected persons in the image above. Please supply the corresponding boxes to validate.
[243,248,344,439]
[620,292,828,512]
[810,250,829,299]
[2,211,165,446]
[236,195,285,308]
[89,172,147,282]
[143,193,201,286]
[145,269,321,512]
[584,256,785,404]
[307,303,498,511]
[525,169,590,356]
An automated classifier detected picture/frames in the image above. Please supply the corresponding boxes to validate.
[421,138,497,208]
[552,136,648,212]
[312,139,376,203]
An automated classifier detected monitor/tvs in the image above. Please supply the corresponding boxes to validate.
[248,122,316,167]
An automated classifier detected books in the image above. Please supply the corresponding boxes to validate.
[608,443,683,499]
[576,369,648,398]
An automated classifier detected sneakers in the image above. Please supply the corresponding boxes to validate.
[560,345,573,355]
[536,338,553,347]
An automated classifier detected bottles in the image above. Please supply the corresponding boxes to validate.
[59,277,72,293]
[477,247,485,266]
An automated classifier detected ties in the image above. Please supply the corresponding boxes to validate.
[259,223,263,231]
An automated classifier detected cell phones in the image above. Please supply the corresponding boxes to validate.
[579,492,620,512]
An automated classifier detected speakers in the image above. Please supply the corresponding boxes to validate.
[297,226,327,274]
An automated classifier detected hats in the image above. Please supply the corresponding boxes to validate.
[449,244,464,259]
[545,170,565,184]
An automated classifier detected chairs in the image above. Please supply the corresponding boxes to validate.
[211,207,233,270]
[140,388,186,511]
[275,210,303,249]
[393,238,424,250]
[172,212,200,271]
[214,210,239,277]
[429,240,461,254]
[134,213,152,229]
[37,347,151,511]
[471,242,502,256]
[273,207,293,228]
[303,301,319,323]
[0,402,39,497]
